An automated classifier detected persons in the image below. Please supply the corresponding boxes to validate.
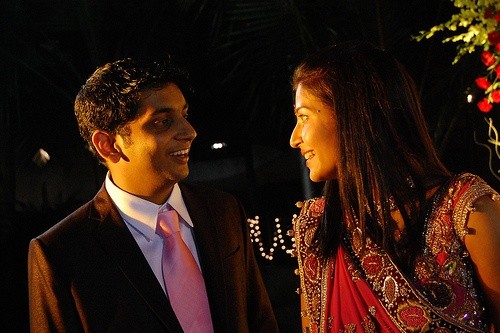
[292,43,500,333]
[28,58,279,333]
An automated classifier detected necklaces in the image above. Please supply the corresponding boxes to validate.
[367,176,413,215]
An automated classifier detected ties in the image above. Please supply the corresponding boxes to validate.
[156,210,214,333]
